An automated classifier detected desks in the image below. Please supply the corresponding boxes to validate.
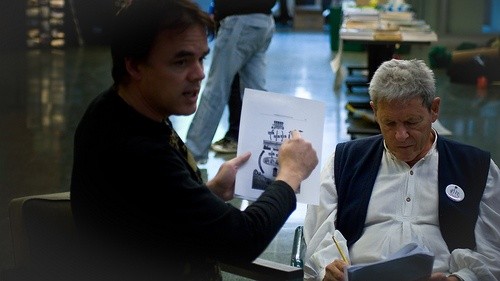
[339,14,437,140]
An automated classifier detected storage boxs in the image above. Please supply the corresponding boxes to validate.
[292,7,321,28]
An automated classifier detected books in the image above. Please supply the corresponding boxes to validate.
[345,11,429,33]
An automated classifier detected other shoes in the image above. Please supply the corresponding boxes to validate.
[213,137,237,153]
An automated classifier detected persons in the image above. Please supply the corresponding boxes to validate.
[303,59,500,281]
[186,0,277,163]
[71,0,318,281]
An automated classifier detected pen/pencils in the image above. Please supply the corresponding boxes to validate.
[331,234,351,268]
